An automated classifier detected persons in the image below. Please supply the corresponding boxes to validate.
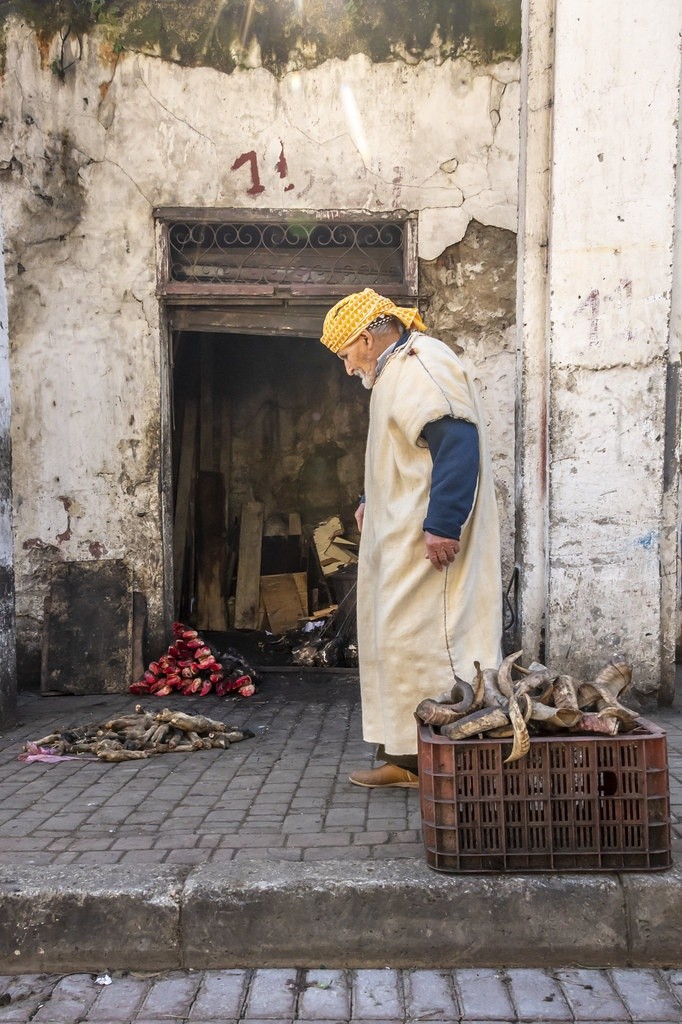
[319,288,503,787]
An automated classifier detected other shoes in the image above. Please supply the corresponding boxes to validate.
[353,762,419,787]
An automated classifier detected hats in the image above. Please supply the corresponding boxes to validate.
[319,287,426,355]
